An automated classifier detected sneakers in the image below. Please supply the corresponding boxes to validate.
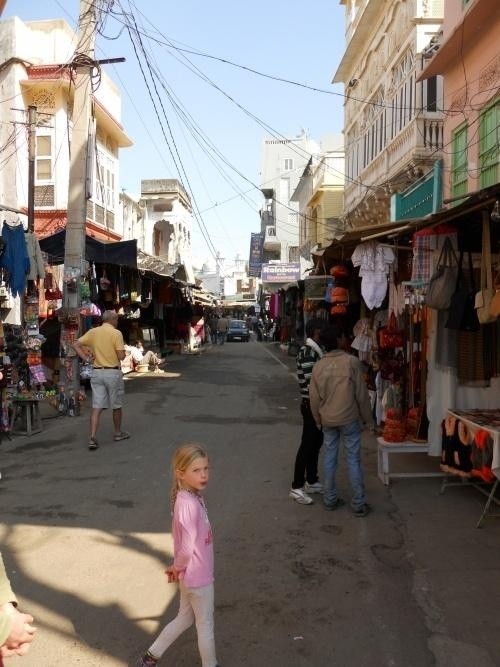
[325,497,345,510]
[287,488,314,505]
[352,502,371,518]
[114,431,130,441]
[88,436,99,450]
[304,480,325,495]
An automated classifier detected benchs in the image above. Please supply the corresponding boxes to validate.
[376,436,462,486]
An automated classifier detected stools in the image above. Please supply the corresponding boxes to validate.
[9,402,42,436]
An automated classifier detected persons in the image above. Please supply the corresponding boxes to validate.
[309,326,376,517]
[139,443,220,667]
[72,310,131,449]
[209,314,219,344]
[205,320,210,343]
[289,318,323,504]
[232,309,302,343]
[217,314,229,345]
[0,550,36,665]
[131,340,168,374]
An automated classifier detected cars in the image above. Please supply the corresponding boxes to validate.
[226,319,250,342]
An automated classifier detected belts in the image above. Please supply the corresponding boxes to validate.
[92,366,122,371]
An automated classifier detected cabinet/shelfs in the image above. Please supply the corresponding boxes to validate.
[441,407,500,526]
